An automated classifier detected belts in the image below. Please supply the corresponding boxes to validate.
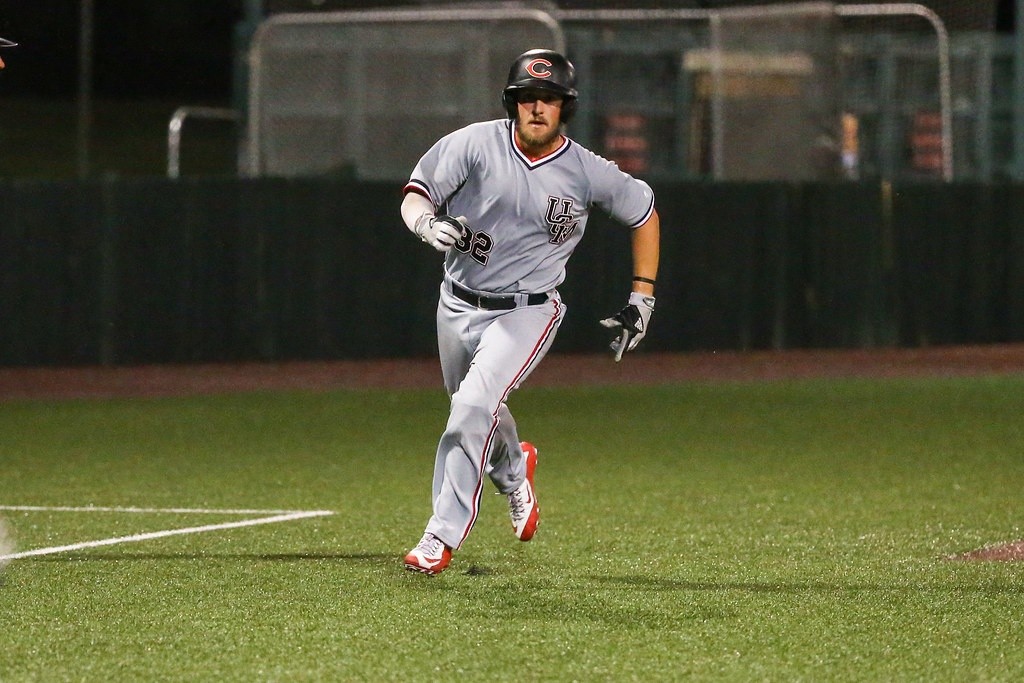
[452,283,547,311]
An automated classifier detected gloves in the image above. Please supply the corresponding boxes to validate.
[600,291,656,362]
[417,214,467,252]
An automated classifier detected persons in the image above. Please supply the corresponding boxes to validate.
[401,49,660,572]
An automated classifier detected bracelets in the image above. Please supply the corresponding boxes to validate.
[632,274,657,286]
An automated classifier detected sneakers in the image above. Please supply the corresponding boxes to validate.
[404,532,452,577]
[494,442,541,542]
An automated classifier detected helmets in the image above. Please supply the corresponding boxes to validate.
[502,49,578,124]
